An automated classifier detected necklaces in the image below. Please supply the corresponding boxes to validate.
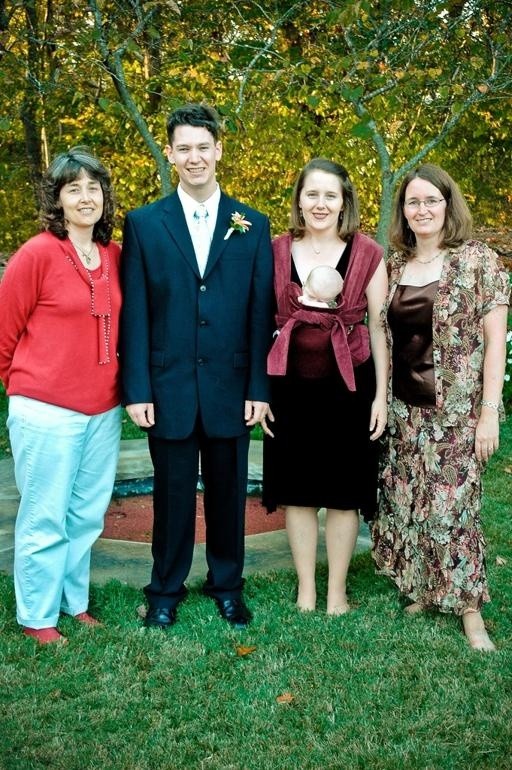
[302,233,343,259]
[411,243,447,265]
[68,239,98,267]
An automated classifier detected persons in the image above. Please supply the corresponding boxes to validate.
[119,102,279,630]
[258,159,390,618]
[2,148,123,646]
[366,162,510,659]
[296,266,344,311]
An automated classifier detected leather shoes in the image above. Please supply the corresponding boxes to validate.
[218,598,254,626]
[143,607,178,630]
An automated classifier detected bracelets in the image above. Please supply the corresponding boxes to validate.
[480,398,501,412]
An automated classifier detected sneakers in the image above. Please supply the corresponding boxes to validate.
[22,627,67,645]
[403,602,423,615]
[466,637,495,651]
[75,611,101,626]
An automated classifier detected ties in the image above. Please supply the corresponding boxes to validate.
[191,205,212,279]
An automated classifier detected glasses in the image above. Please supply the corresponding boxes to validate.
[403,197,446,208]
